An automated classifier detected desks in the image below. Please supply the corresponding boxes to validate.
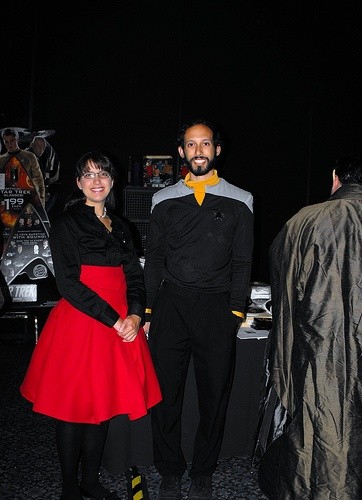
[100,282,271,475]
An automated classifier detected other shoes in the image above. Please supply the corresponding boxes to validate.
[188,475,212,500]
[157,475,181,500]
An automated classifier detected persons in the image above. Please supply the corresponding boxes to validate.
[25,137,45,180]
[142,120,253,500]
[20,153,162,500]
[0,128,45,210]
[252,153,362,500]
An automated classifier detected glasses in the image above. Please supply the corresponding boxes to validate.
[81,170,112,179]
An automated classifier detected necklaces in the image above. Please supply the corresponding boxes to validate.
[97,207,107,218]
